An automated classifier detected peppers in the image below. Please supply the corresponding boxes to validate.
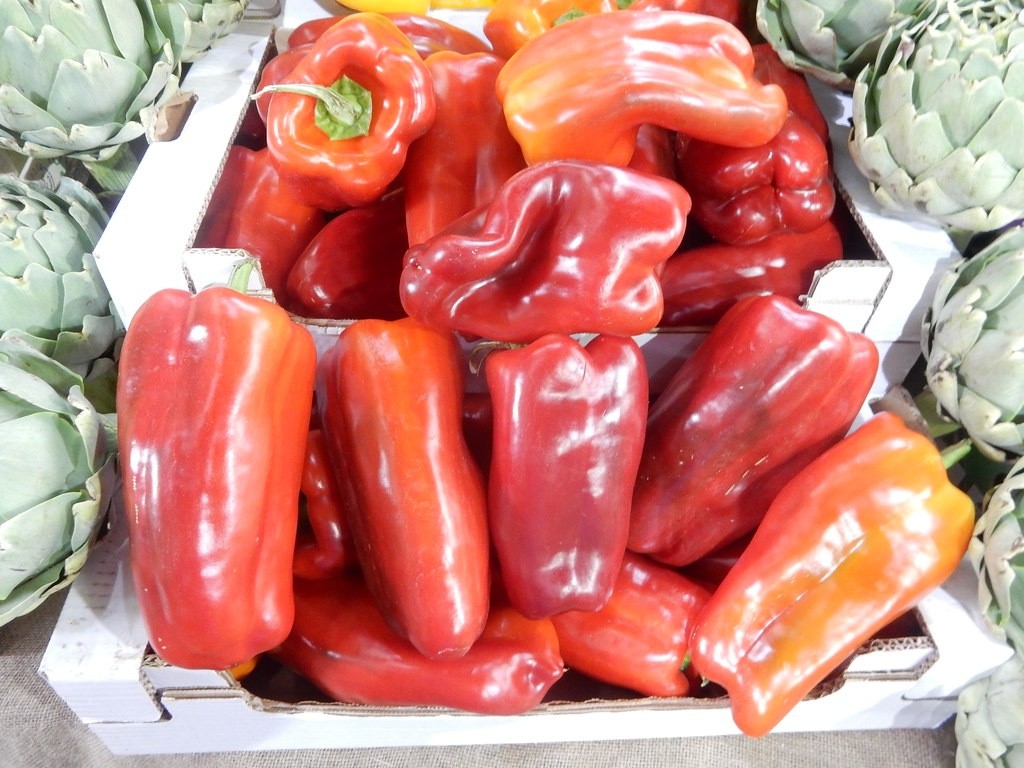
[116,0,975,736]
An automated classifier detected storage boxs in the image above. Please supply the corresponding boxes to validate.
[38,0,1017,756]
